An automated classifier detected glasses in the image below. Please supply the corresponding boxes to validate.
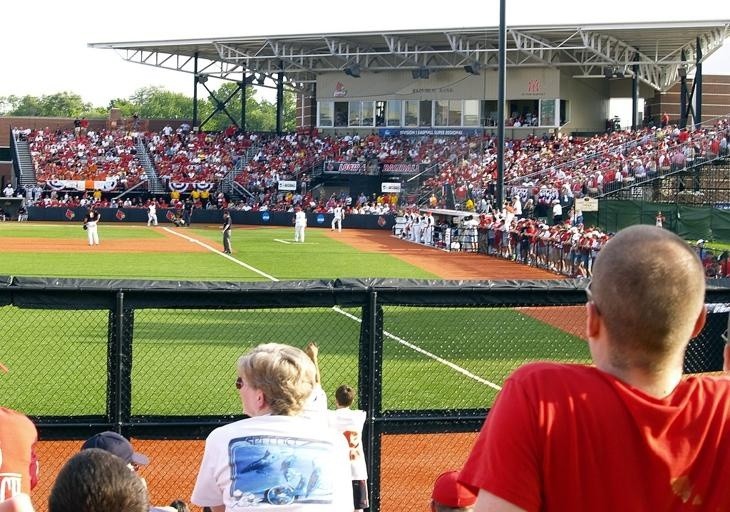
[584,278,601,316]
[129,462,140,472]
[235,377,246,389]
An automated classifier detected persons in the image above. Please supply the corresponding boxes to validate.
[453,223,730,511]
[167,499,191,512]
[78,429,180,511]
[292,206,308,244]
[44,446,153,511]
[0,399,40,511]
[302,341,371,511]
[189,341,358,511]
[221,210,233,254]
[84,205,102,247]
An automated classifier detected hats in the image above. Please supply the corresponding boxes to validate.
[79,431,151,469]
